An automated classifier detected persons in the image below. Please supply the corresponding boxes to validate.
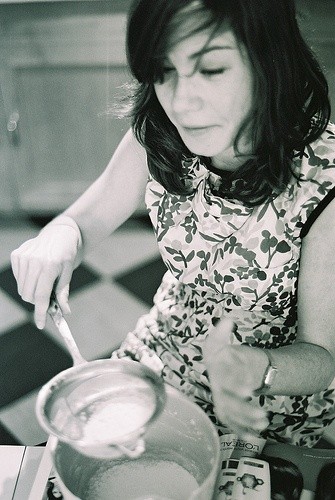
[11,0,335,451]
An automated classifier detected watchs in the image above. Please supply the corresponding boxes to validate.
[254,350,278,397]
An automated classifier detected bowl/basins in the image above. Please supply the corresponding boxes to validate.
[44,390,220,500]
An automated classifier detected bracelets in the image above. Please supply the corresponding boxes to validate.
[49,214,84,272]
